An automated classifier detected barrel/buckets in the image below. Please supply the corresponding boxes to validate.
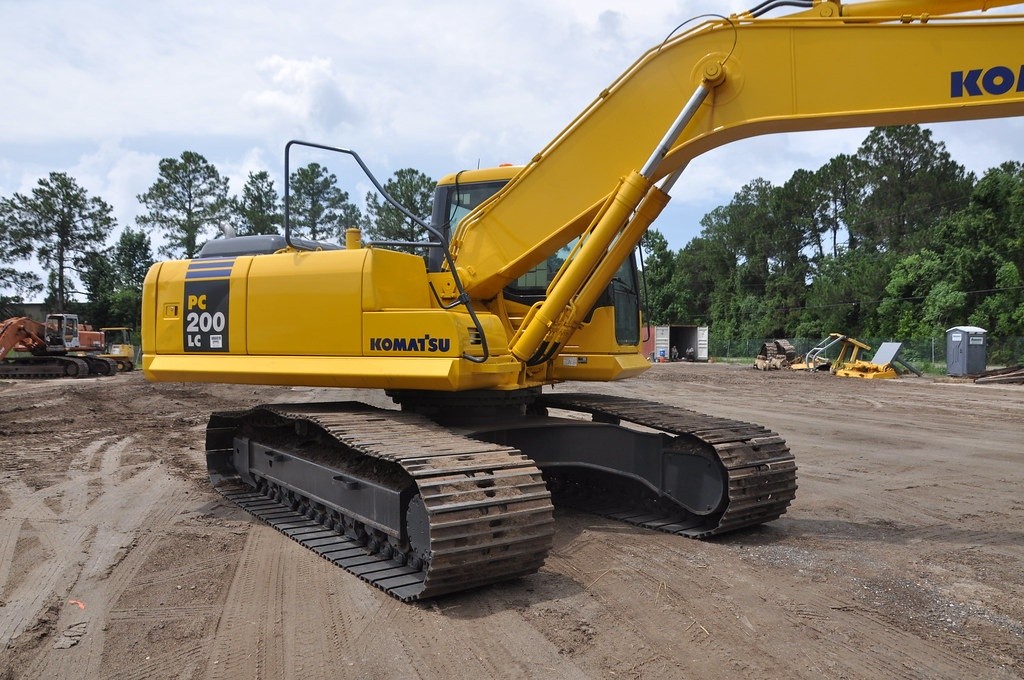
[659,349,665,356]
[660,356,665,363]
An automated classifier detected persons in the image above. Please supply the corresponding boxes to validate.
[686,345,695,362]
[671,345,678,361]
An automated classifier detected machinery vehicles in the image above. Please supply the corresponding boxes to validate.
[141,0,1022,614]
[0,313,136,380]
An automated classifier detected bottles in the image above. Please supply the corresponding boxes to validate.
[656,357,658,363]
